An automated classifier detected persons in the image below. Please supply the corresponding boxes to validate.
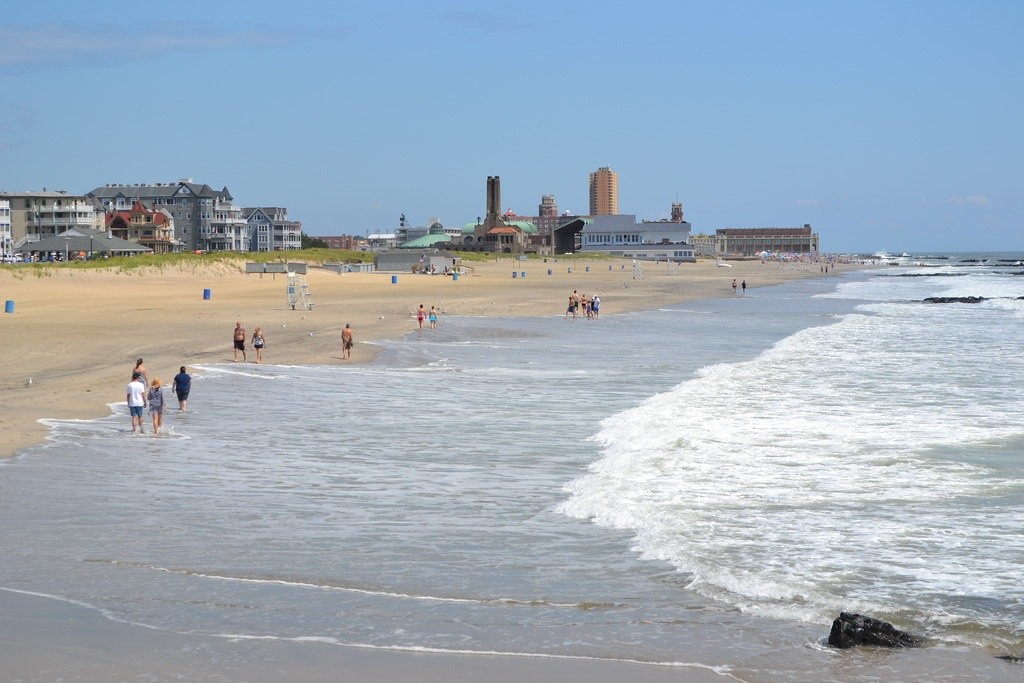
[340,323,353,360]
[171,366,191,413]
[147,377,166,434]
[565,297,576,319]
[731,279,737,292]
[572,290,579,310]
[132,358,149,407]
[821,266,823,274]
[126,373,147,434]
[417,304,427,331]
[580,294,588,314]
[250,327,266,363]
[233,321,248,362]
[824,266,828,273]
[591,295,600,318]
[586,301,594,320]
[742,280,747,292]
[428,306,438,332]
[430,264,434,273]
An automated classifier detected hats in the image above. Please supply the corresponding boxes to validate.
[594,295,597,298]
[149,378,161,389]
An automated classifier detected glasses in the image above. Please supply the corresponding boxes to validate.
[237,324,240,325]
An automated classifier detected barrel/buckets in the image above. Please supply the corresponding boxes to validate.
[6,301,14,312]
[609,266,612,270]
[522,272,525,277]
[453,274,457,280]
[586,267,590,272]
[204,289,210,299]
[548,269,552,275]
[513,272,517,278]
[392,276,397,283]
[568,267,572,273]
